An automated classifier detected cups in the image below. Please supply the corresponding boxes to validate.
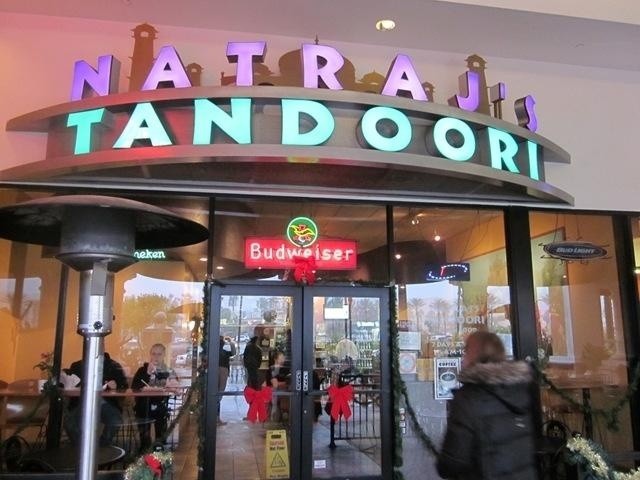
[439,371,456,391]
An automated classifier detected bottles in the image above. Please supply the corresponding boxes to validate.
[320,323,375,369]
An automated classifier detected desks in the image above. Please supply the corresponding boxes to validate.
[29,444,126,473]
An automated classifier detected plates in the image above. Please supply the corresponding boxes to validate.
[140,385,167,392]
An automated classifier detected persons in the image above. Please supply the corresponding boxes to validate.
[435,331,538,480]
[215,323,291,427]
[130,344,178,452]
[63,351,128,445]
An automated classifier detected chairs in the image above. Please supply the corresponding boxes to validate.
[534,419,572,480]
[15,457,58,475]
[99,415,157,470]
[0,434,33,473]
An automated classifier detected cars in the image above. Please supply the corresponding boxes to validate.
[176,347,203,368]
[233,334,251,343]
